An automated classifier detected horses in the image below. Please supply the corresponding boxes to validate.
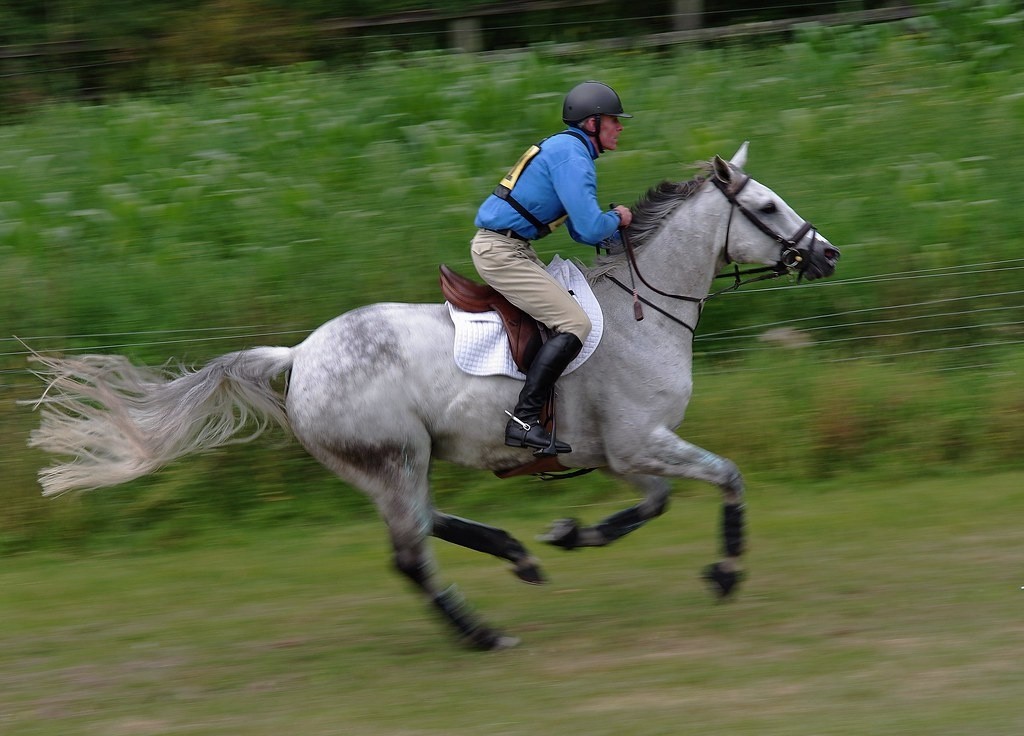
[12,140,842,653]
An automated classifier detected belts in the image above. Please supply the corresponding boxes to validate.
[483,225,529,242]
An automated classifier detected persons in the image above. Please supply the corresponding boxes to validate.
[468,81,633,454]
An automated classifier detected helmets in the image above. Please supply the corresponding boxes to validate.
[562,80,634,122]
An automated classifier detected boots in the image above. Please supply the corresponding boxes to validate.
[504,332,584,452]
